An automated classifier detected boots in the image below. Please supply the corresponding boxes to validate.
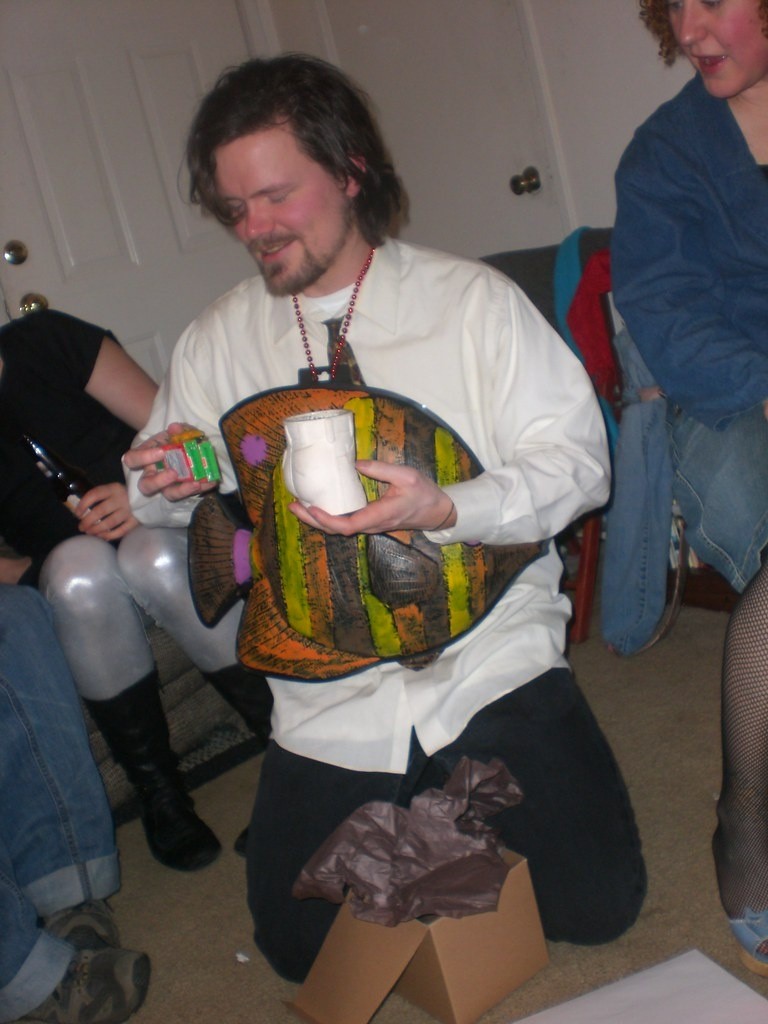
[196,658,274,859]
[81,664,224,873]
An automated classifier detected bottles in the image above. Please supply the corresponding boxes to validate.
[23,434,123,549]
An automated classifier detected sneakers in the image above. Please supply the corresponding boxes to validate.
[43,896,122,947]
[20,949,152,1024]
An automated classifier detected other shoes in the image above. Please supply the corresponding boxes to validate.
[727,905,767,967]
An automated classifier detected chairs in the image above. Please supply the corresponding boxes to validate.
[547,258,746,644]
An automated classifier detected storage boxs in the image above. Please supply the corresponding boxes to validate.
[282,849,548,1024]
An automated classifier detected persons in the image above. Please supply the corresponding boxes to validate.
[1,305,277,873]
[1,582,151,1024]
[612,0,768,959]
[119,52,649,983]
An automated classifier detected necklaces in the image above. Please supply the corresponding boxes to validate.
[291,247,374,391]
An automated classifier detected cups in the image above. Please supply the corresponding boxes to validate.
[283,410,366,516]
